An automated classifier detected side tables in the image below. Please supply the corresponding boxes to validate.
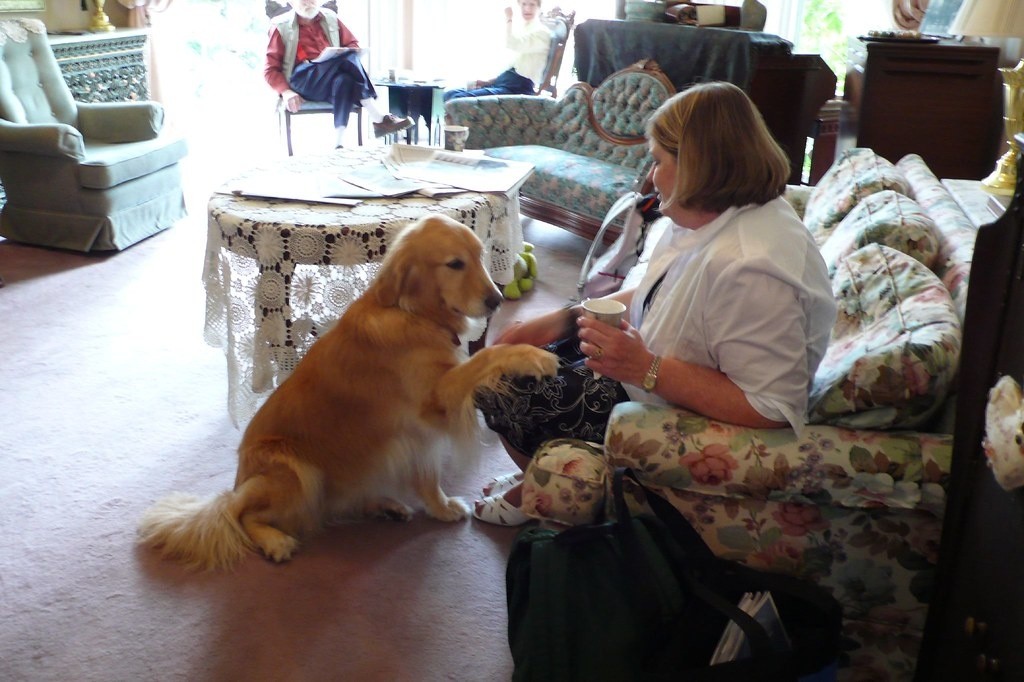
[940,177,997,228]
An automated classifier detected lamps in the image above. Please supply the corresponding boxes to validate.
[949,0,1024,197]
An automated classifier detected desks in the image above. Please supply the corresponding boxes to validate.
[856,40,1003,181]
[374,75,448,147]
[47,24,152,103]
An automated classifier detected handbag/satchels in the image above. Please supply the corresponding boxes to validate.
[574,191,645,301]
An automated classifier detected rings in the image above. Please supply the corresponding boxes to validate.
[594,347,603,358]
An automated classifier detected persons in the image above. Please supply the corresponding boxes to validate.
[263,0,415,150]
[469,82,838,528]
[442,0,552,104]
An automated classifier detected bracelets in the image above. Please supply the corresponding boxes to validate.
[506,19,512,24]
[564,303,582,336]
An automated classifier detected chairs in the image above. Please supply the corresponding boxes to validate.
[264,0,365,157]
[538,6,576,98]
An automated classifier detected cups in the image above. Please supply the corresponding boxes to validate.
[444,125,470,153]
[581,298,627,329]
[388,69,399,83]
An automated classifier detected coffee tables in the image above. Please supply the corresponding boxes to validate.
[200,188,524,431]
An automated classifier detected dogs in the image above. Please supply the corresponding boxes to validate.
[137,214,565,571]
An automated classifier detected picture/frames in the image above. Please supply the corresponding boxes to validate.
[0,0,47,13]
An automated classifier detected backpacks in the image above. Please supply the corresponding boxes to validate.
[506,466,842,682]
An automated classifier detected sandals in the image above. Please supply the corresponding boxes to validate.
[473,491,533,526]
[481,473,519,497]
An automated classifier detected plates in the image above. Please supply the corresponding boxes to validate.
[857,34,940,45]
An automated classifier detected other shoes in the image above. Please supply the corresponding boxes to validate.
[373,114,415,138]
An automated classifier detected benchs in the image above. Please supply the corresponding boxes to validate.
[443,55,678,247]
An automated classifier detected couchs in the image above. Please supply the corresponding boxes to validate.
[520,146,977,682]
[0,16,190,256]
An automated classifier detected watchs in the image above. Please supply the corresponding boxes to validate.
[642,355,661,392]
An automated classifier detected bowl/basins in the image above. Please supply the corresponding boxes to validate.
[624,0,666,21]
